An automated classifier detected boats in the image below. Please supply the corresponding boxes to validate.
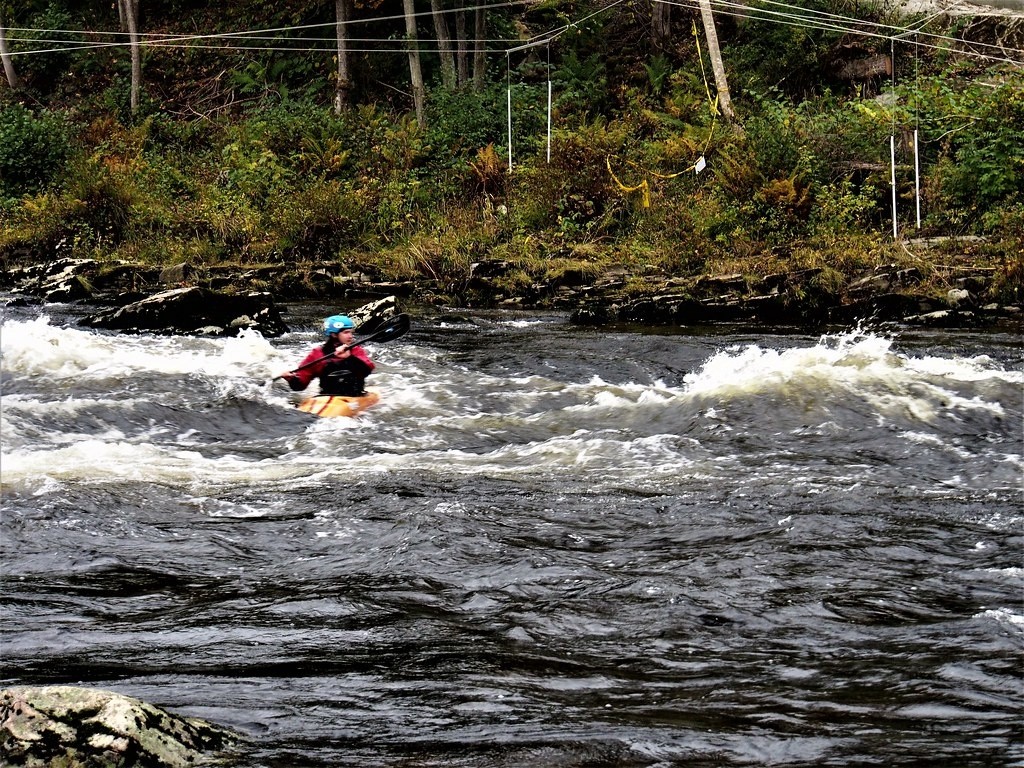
[299,390,379,419]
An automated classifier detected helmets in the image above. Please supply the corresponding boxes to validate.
[324,315,355,336]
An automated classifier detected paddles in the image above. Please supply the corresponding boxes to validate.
[261,312,412,385]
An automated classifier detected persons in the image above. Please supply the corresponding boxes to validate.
[271,315,376,399]
